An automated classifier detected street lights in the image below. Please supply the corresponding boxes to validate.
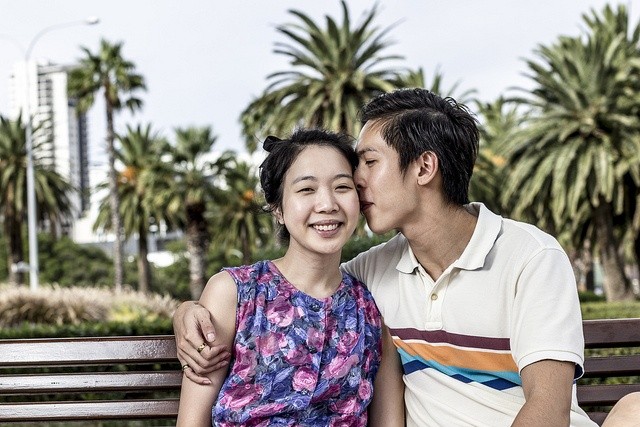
[24,15,104,288]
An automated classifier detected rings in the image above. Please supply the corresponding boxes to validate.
[182,365,189,371]
[197,343,207,353]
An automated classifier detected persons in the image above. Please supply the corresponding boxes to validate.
[174,129,406,427]
[171,88,601,427]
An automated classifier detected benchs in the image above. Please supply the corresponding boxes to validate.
[0,318,640,427]
